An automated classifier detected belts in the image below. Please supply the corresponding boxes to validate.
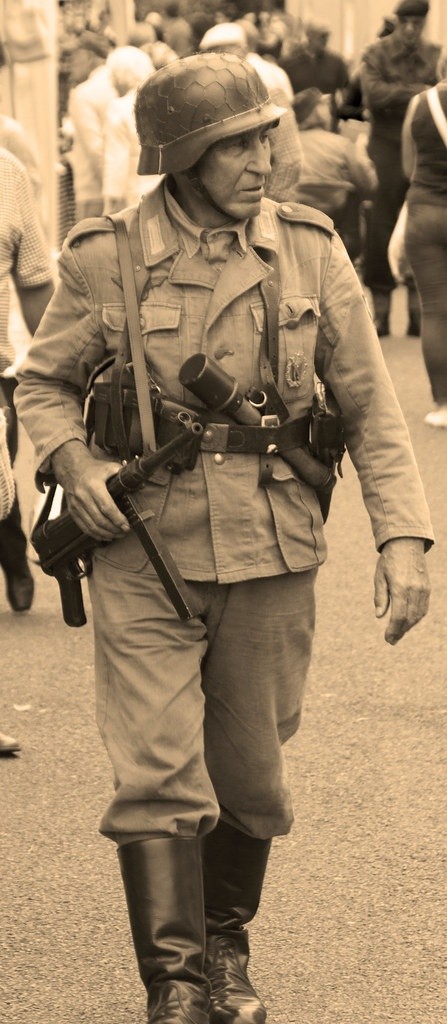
[86,396,311,454]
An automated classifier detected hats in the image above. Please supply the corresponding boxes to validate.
[395,0,428,15]
[291,87,331,123]
[199,23,245,49]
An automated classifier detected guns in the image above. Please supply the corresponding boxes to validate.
[27,409,204,630]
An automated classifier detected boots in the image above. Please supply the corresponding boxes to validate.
[115,818,272,1024]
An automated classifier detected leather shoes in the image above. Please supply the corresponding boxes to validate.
[6,561,34,610]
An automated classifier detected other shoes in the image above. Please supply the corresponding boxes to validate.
[426,412,447,427]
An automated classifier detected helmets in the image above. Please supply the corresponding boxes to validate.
[133,51,289,178]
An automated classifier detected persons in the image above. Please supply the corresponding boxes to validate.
[333,1,447,431]
[12,52,433,1024]
[0,0,379,753]
